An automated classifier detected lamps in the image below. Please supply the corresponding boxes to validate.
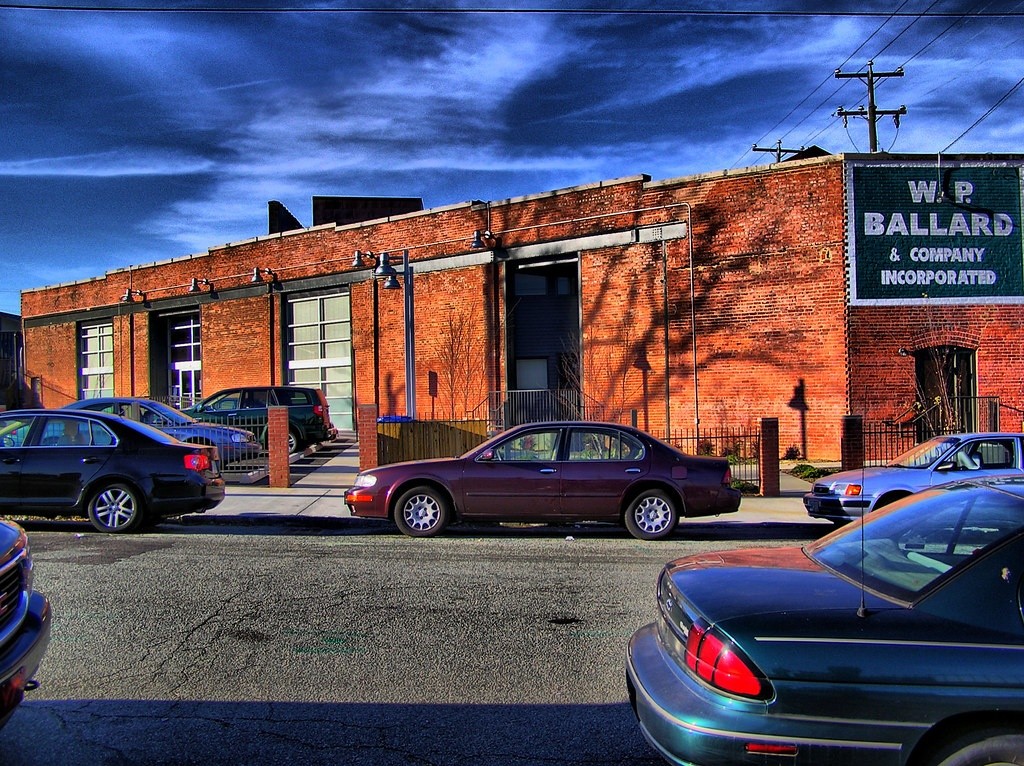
[189,277,208,292]
[936,151,944,203]
[122,288,142,302]
[351,249,373,267]
[472,229,491,248]
[251,267,271,282]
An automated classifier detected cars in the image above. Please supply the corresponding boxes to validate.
[805,432,1024,527]
[343,421,741,540]
[0,398,260,472]
[0,409,224,534]
[625,476,1024,766]
[144,387,338,456]
[0,515,52,731]
[0,421,32,447]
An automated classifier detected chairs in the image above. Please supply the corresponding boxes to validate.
[970,452,983,470]
[144,411,154,424]
[57,422,85,446]
